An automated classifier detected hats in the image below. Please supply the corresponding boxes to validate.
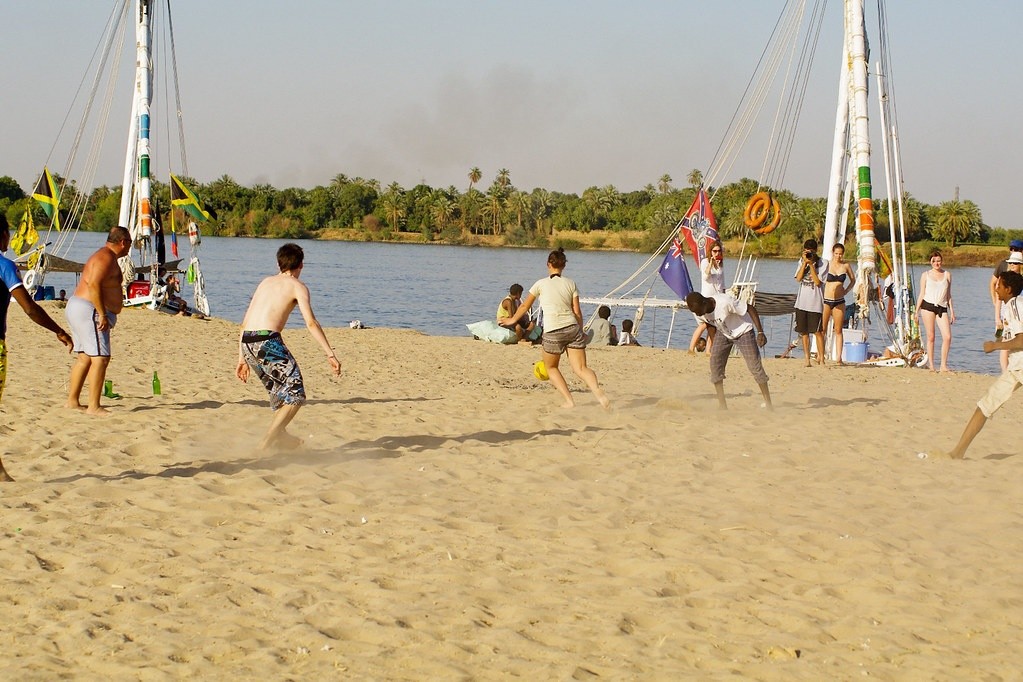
[1008,240,1023,250]
[1006,252,1023,263]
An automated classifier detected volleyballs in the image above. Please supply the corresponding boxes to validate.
[534,360,549,381]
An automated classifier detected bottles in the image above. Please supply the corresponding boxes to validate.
[105,381,113,397]
[153,371,160,394]
[103,393,119,397]
[848,316,853,329]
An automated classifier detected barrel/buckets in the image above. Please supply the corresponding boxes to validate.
[34,285,44,300]
[44,286,55,300]
[845,342,871,362]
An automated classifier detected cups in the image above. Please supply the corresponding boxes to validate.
[175,278,180,283]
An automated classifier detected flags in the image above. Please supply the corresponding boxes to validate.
[680,187,723,268]
[170,173,218,224]
[659,237,693,300]
[32,167,68,232]
[11,206,39,257]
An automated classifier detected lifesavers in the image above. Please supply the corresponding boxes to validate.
[23,270,37,289]
[743,191,781,234]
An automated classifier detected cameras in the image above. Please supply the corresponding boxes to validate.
[805,251,814,260]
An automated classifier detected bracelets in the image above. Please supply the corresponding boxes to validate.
[757,332,765,334]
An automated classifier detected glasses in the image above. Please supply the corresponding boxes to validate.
[1009,262,1018,265]
[1010,247,1018,251]
[120,238,132,244]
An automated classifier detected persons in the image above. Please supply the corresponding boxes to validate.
[499,247,609,412]
[56,289,68,301]
[496,284,534,343]
[690,241,725,353]
[617,319,639,346]
[586,306,613,346]
[687,293,772,407]
[235,241,341,450]
[0,212,74,483]
[65,226,132,415]
[794,240,856,367]
[915,250,955,372]
[952,239,1023,459]
[161,275,187,316]
[158,267,167,286]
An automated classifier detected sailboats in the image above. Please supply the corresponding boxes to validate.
[464,0,933,368]
[2,1,187,300]
